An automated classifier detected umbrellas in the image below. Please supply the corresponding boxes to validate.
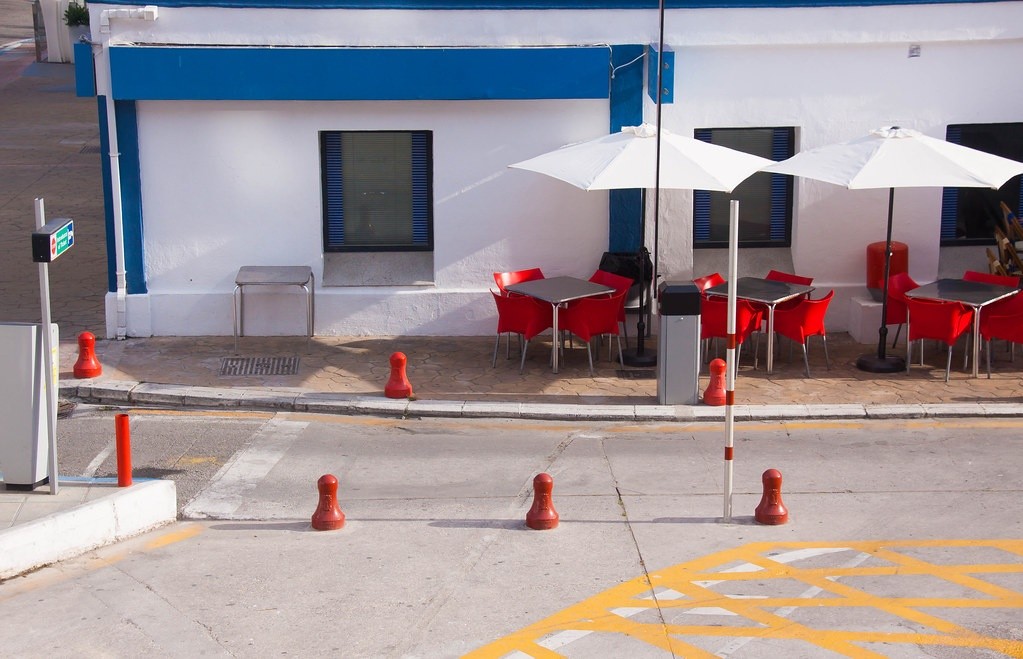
[506,121,779,367]
[757,126,1023,373]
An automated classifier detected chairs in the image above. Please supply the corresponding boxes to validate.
[995,225,1023,279]
[690,268,835,379]
[999,201,1023,254]
[985,247,1007,275]
[880,269,1023,383]
[489,246,653,379]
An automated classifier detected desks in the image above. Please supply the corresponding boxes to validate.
[232,265,316,356]
[505,276,617,374]
[904,278,1021,379]
[703,276,816,377]
